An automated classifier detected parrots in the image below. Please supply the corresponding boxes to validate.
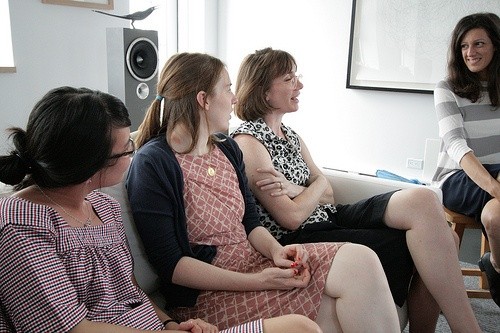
[91,7,156,29]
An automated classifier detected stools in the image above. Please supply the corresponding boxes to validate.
[441,204,491,299]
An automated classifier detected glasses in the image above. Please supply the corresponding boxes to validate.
[271,74,303,90]
[107,138,136,160]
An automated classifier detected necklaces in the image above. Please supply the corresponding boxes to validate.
[169,133,218,177]
[35,181,92,228]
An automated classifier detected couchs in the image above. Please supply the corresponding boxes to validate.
[0,169,443,333]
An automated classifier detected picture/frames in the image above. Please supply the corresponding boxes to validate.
[347,0,500,94]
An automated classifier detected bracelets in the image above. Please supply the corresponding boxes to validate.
[163,319,176,327]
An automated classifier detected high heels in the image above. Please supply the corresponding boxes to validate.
[478,251,500,308]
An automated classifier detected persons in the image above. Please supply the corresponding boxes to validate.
[125,52,402,333]
[0,85,323,333]
[228,46,484,333]
[433,12,500,308]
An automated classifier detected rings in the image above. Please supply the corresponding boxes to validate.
[280,182,284,189]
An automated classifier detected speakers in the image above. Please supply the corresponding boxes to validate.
[106,27,158,133]
